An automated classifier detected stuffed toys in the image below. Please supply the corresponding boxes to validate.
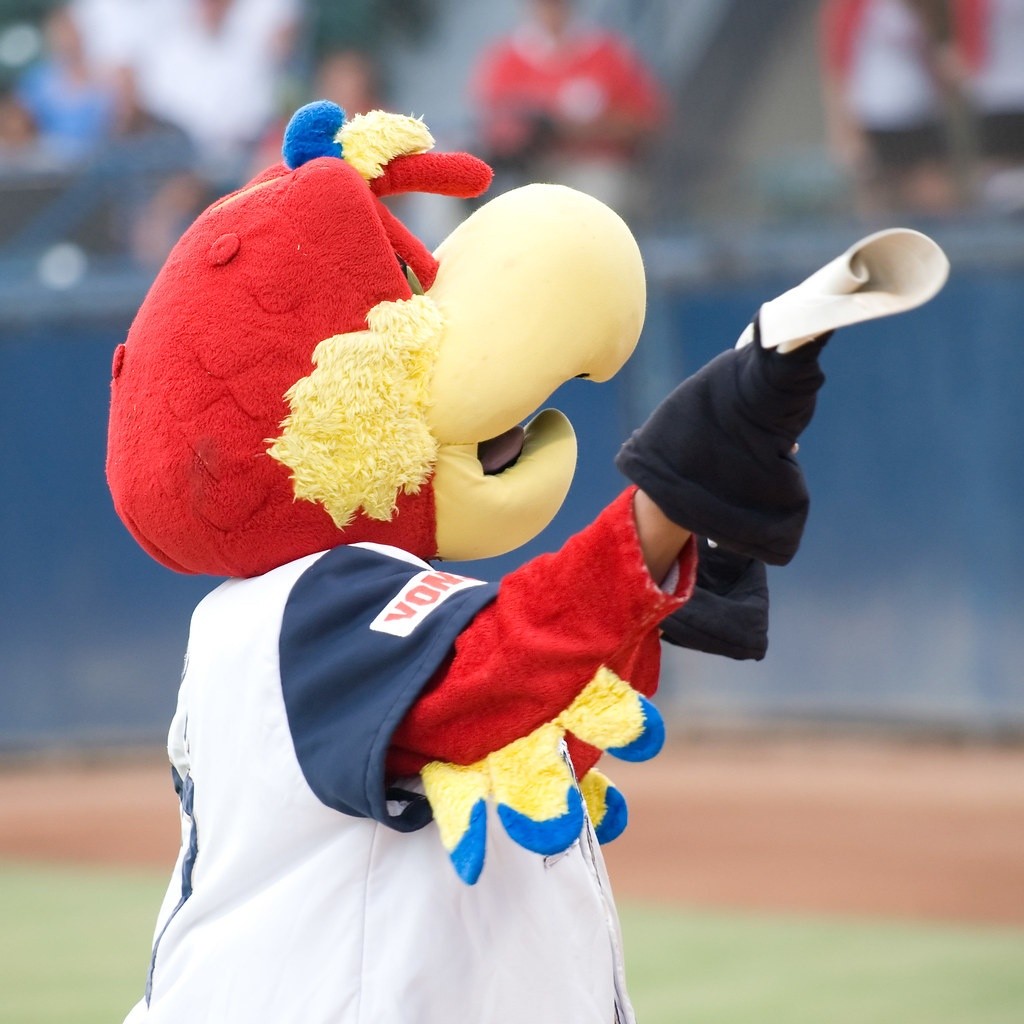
[104,102,835,1024]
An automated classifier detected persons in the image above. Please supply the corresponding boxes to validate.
[0,2,1024,300]
